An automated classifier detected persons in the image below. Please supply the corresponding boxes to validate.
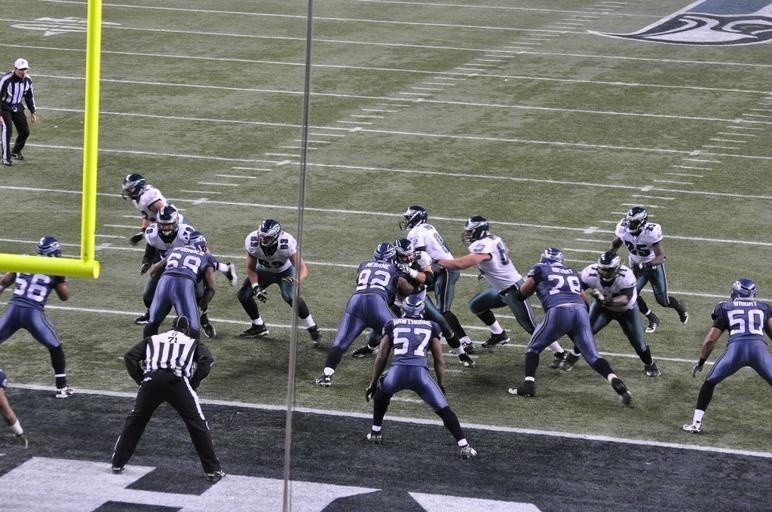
[0,370,28,448]
[559,251,663,377]
[237,219,323,349]
[1,236,74,399]
[609,207,689,334]
[122,170,238,342]
[0,57,37,166]
[314,204,478,458]
[431,216,567,369]
[111,314,225,483]
[682,279,772,434]
[507,248,636,409]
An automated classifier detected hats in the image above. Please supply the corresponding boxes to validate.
[14,58,30,70]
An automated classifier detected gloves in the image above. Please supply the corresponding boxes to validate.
[11,431,28,448]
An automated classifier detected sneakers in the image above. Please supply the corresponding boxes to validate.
[683,421,703,433]
[239,323,269,338]
[55,386,73,398]
[459,443,477,459]
[112,464,125,473]
[449,329,510,368]
[133,310,150,325]
[307,325,323,345]
[0,158,13,164]
[507,386,535,397]
[366,430,382,444]
[643,359,662,376]
[311,374,332,387]
[201,317,216,338]
[352,344,378,358]
[676,300,689,324]
[550,351,581,371]
[205,471,225,482]
[612,377,632,406]
[645,316,659,333]
[9,151,24,160]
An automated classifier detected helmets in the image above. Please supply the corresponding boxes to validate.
[597,251,622,282]
[373,238,415,268]
[399,205,427,232]
[156,205,179,236]
[37,237,61,258]
[730,279,757,299]
[258,220,280,248]
[120,174,146,201]
[540,248,565,264]
[624,206,648,233]
[461,215,490,246]
[399,297,427,318]
[188,231,208,252]
